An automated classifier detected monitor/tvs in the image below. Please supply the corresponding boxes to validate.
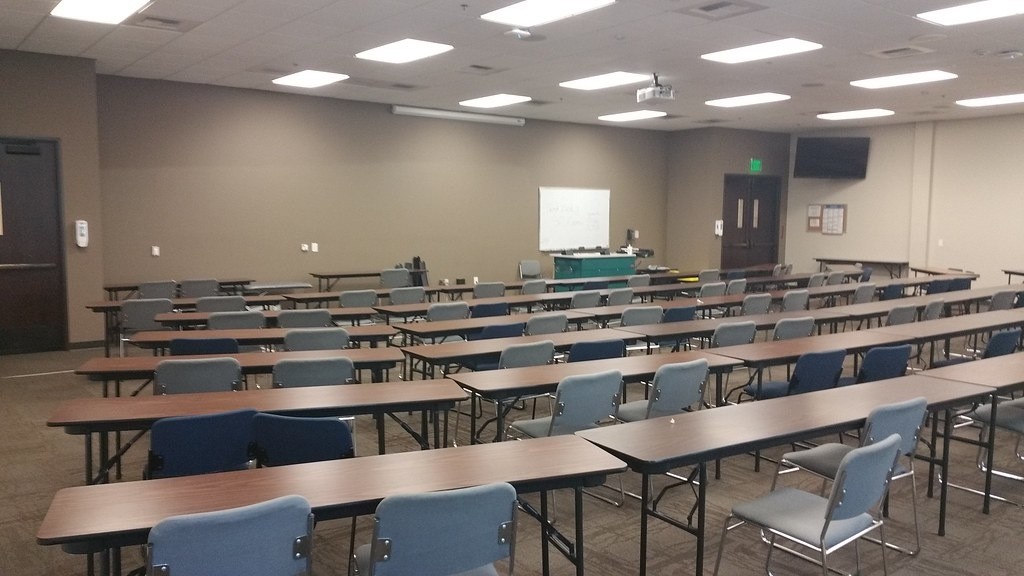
[794,137,870,179]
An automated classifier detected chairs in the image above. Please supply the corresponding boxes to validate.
[36,256,1024,573]
